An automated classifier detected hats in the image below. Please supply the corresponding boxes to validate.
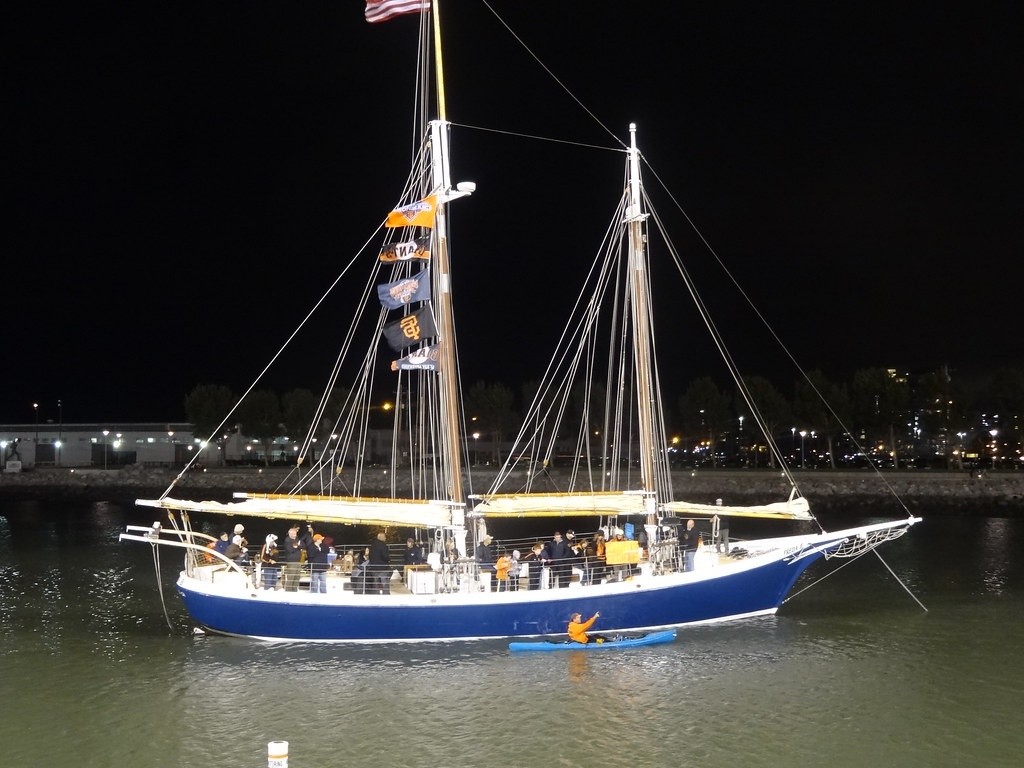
[571,613,581,621]
[597,530,604,536]
[407,538,414,543]
[234,524,244,532]
[554,532,561,535]
[715,498,723,505]
[266,534,278,554]
[313,534,325,541]
[567,529,575,537]
[483,534,493,540]
[232,535,248,553]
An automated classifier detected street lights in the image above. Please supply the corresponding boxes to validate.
[472,431,480,466]
[167,430,175,474]
[799,430,807,469]
[957,432,967,452]
[101,428,109,470]
[988,429,998,471]
[790,426,797,469]
[116,431,122,467]
[32,402,39,443]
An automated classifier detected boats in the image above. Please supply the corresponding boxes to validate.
[509,628,678,653]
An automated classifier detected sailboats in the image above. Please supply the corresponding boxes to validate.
[115,0,929,643]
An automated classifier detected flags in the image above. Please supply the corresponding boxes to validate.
[365,0,431,22]
[386,195,436,228]
[391,343,440,371]
[380,232,431,265]
[383,306,437,352]
[378,268,429,310]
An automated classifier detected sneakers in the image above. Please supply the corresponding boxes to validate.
[717,550,721,556]
[613,633,620,641]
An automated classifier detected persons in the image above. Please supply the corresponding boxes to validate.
[610,530,628,582]
[548,532,571,585]
[561,529,588,567]
[567,611,618,643]
[405,538,422,565]
[440,540,459,570]
[357,533,390,594]
[215,524,249,569]
[683,520,700,571]
[261,527,329,593]
[476,535,496,586]
[496,552,512,592]
[716,498,729,555]
[590,530,606,584]
[523,541,548,590]
